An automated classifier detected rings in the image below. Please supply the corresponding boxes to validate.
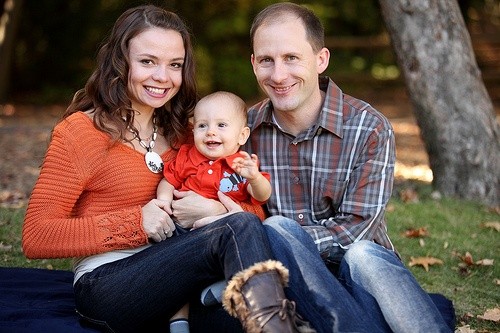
[164,228,171,234]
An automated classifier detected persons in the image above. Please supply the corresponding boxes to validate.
[21,6,315,333]
[189,2,453,333]
[157,91,271,333]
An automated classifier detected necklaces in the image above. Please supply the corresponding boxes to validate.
[120,109,164,173]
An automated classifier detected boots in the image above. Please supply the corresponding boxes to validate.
[224,259,301,333]
